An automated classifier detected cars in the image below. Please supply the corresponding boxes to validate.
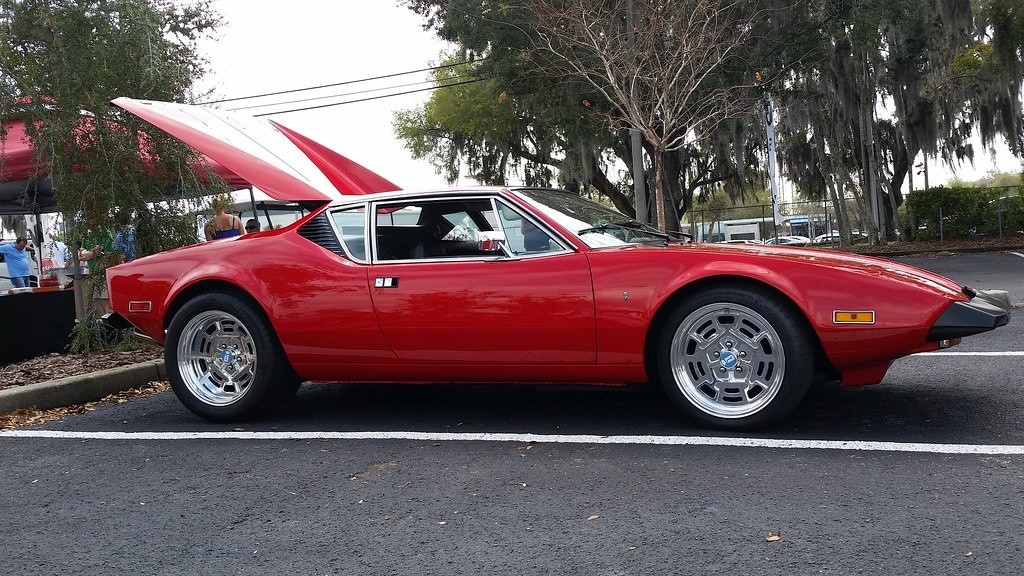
[106,186,1012,436]
[727,232,866,245]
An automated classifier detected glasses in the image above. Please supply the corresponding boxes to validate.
[245,225,257,230]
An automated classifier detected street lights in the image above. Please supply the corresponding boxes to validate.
[915,162,929,191]
[465,175,485,218]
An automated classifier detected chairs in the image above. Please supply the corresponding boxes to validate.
[409,240,478,258]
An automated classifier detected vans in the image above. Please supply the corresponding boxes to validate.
[0,239,73,292]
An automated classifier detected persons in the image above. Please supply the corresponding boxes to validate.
[80,224,118,315]
[112,210,137,264]
[246,219,260,233]
[204,195,245,241]
[0,237,35,288]
[520,218,551,250]
